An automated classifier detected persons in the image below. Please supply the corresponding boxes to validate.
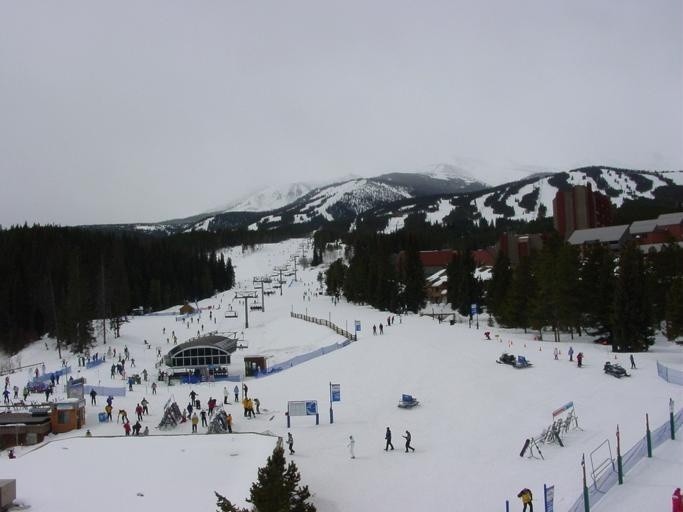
[567,346,574,361]
[383,426,394,452]
[345,434,356,459]
[0,367,60,403]
[629,353,638,370]
[401,430,414,453]
[285,431,295,456]
[552,347,559,360]
[76,310,217,435]
[372,315,395,335]
[576,351,584,368]
[223,384,261,435]
[516,487,534,512]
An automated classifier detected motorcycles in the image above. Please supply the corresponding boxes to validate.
[604,361,631,379]
[26,381,50,393]
[398,394,420,409]
[495,353,532,368]
